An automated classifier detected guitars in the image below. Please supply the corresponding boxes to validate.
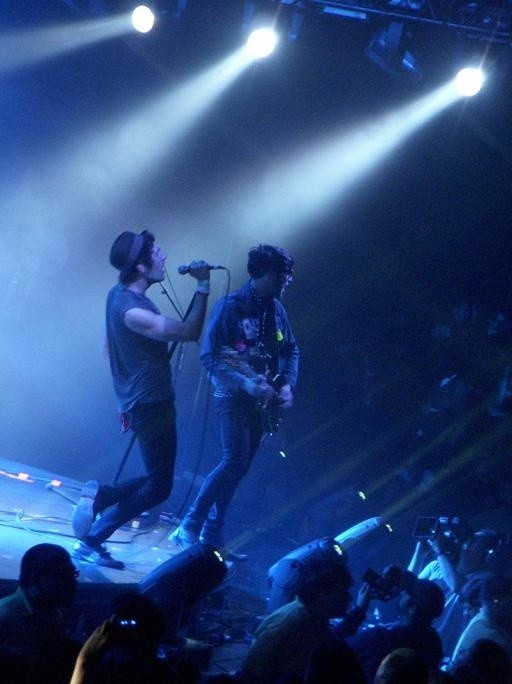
[221,344,285,435]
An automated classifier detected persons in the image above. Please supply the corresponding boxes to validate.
[233,532,510,682]
[68,226,215,570]
[166,239,301,559]
[0,543,164,683]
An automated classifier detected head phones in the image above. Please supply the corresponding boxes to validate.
[485,533,504,565]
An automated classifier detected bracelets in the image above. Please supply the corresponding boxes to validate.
[194,278,212,296]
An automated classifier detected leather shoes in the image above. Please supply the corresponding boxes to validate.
[220,546,247,561]
[169,525,201,552]
[73,540,125,568]
[72,479,102,539]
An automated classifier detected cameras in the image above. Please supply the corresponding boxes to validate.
[363,565,405,600]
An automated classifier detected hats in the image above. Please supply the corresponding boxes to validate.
[109,229,147,279]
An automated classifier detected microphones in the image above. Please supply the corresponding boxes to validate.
[178,263,223,276]
